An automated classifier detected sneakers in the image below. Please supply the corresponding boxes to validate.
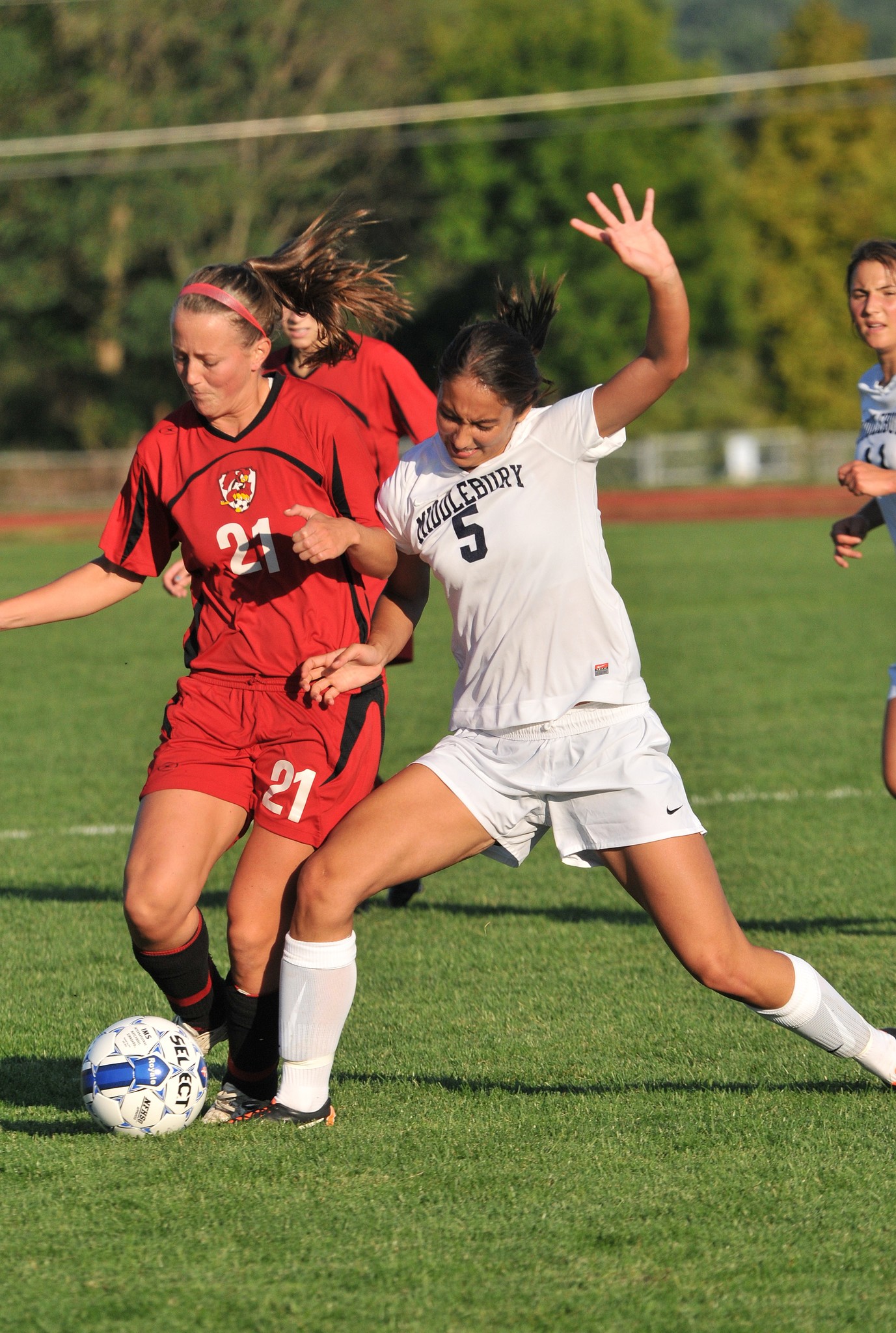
[203,1082,275,1124]
[226,1098,335,1129]
[171,1013,232,1054]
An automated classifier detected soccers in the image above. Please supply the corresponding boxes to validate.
[79,1017,209,1136]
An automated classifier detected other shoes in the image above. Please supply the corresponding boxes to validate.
[388,878,420,908]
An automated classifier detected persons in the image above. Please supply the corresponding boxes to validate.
[830,240,896,802]
[0,196,437,1125]
[222,183,896,1128]
